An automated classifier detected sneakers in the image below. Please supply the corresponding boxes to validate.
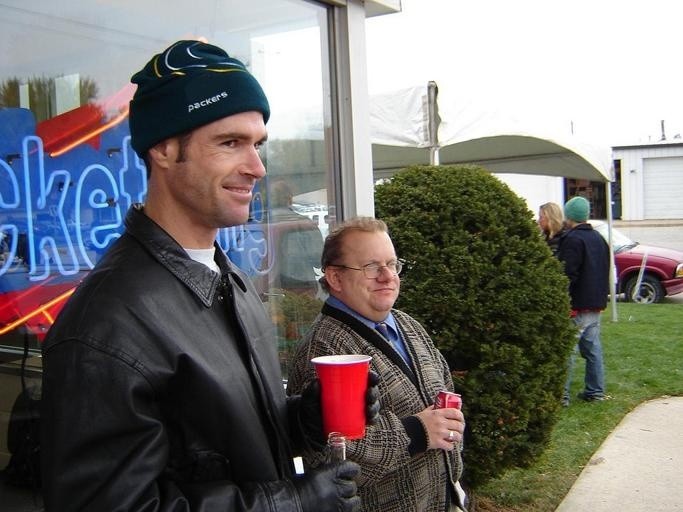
[577,392,603,402]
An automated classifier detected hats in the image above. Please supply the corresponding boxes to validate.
[129,39,270,154]
[564,196,590,223]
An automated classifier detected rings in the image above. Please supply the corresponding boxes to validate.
[447,430,453,441]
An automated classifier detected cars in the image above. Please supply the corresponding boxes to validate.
[585,219,683,305]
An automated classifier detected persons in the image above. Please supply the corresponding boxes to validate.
[264,176,330,306]
[538,202,571,259]
[555,196,610,406]
[284,218,469,511]
[31,40,386,510]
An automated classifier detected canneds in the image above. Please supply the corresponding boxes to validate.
[432,390,463,446]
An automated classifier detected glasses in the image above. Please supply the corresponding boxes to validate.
[323,258,405,278]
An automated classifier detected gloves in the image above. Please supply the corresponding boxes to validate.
[292,461,361,512]
[300,369,380,438]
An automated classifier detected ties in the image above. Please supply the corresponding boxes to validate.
[376,322,389,340]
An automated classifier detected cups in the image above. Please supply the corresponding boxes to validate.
[310,354,372,440]
